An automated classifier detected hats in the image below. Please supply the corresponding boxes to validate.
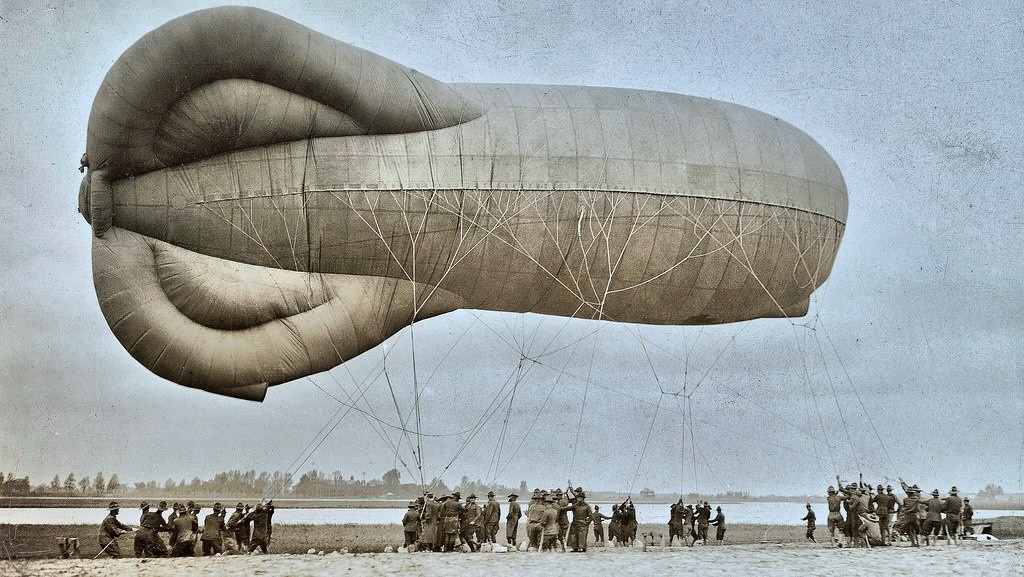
[805,503,811,507]
[532,487,588,501]
[236,503,244,509]
[827,483,940,496]
[866,513,880,521]
[685,503,723,511]
[244,503,252,508]
[963,497,969,501]
[106,502,120,511]
[508,493,519,497]
[138,501,152,510]
[612,504,635,511]
[212,502,222,511]
[407,491,496,507]
[172,500,203,512]
[256,504,263,510]
[948,487,960,494]
[594,505,599,510]
[157,500,167,511]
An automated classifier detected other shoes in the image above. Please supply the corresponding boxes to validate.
[550,548,586,552]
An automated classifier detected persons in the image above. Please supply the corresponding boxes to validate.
[592,504,613,547]
[668,499,726,546]
[98,501,201,558]
[200,500,274,556]
[526,487,593,552]
[402,491,501,553]
[828,473,975,547]
[505,493,522,547]
[608,498,638,548]
[801,502,818,543]
[65,538,81,557]
